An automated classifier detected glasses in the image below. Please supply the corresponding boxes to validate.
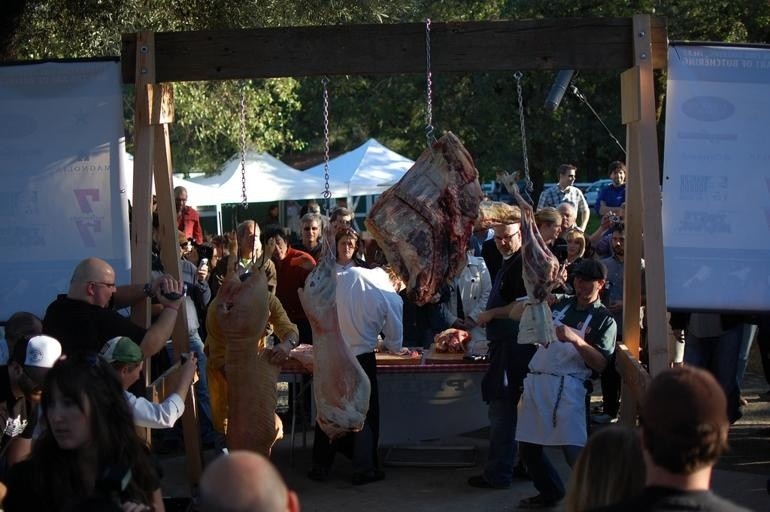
[342,241,354,248]
[493,231,520,245]
[87,279,117,289]
[303,226,319,231]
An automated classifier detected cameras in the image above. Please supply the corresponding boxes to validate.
[180,352,200,372]
[151,282,185,304]
[609,216,620,222]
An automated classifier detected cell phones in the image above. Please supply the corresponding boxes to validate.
[197,258,208,273]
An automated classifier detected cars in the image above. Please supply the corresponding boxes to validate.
[583,179,615,207]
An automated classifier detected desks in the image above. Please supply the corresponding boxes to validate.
[278,348,492,469]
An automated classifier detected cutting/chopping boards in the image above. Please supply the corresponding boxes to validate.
[282,350,424,369]
[425,343,488,365]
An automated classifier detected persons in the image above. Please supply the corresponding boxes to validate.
[584,367,756,512]
[535,165,590,232]
[567,425,648,512]
[40,256,184,367]
[565,229,586,262]
[439,254,491,353]
[558,203,584,259]
[514,260,616,506]
[595,163,627,216]
[264,202,284,228]
[262,227,317,421]
[598,222,646,417]
[95,337,198,428]
[1,311,43,362]
[308,263,404,485]
[293,212,325,264]
[333,228,377,270]
[209,219,277,298]
[469,223,537,488]
[533,206,567,259]
[668,310,759,423]
[329,205,355,236]
[197,451,299,512]
[150,229,226,451]
[156,186,203,247]
[151,194,158,215]
[5,355,169,511]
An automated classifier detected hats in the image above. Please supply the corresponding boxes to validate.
[99,336,144,368]
[572,259,608,281]
[637,366,728,434]
[23,334,64,369]
